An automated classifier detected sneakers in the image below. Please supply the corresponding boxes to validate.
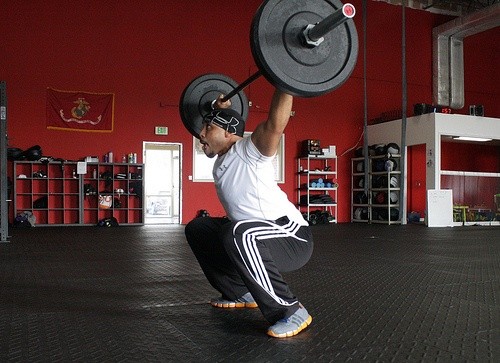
[212,292,258,307]
[267,302,312,338]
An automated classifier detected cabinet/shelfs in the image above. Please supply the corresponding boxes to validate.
[351,154,403,225]
[297,156,337,225]
[14,161,146,227]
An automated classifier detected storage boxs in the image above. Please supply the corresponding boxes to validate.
[302,139,336,157]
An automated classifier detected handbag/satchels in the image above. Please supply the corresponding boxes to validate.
[97,217,118,226]
[13,211,36,228]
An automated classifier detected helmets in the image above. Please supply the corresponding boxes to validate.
[6,144,41,161]
[354,143,400,221]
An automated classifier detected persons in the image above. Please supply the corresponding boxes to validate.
[185,88,311,338]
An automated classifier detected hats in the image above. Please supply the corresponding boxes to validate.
[201,108,245,138]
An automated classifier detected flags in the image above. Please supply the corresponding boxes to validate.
[45,86,116,134]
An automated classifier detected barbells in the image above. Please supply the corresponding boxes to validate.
[178,0,361,140]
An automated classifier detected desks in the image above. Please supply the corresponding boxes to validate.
[453,205,492,222]
